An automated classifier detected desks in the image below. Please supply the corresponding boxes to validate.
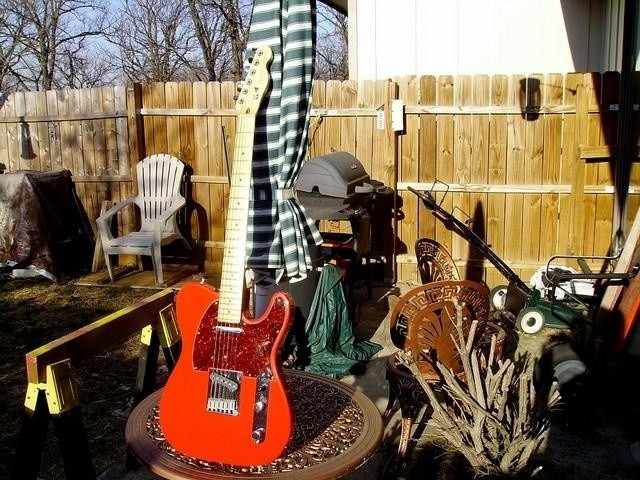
[123,368,386,480]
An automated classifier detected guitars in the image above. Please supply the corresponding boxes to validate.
[159,44,296,467]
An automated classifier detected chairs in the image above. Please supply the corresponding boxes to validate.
[95,154,200,286]
[382,238,507,480]
